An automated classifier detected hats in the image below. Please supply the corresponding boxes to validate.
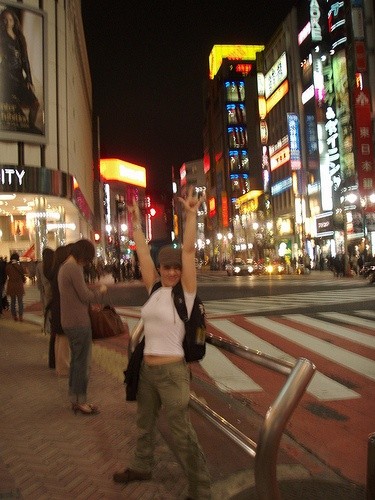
[157,246,183,266]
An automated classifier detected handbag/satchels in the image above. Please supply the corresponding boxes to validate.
[88,285,125,340]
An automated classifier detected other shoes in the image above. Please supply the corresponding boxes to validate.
[112,467,151,482]
[72,401,100,415]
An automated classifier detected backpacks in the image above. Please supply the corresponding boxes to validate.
[150,280,207,363]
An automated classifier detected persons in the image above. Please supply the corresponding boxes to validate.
[0,228,375,312]
[5,253,26,322]
[48,244,71,370]
[112,184,213,500]
[36,247,57,335]
[0,8,41,132]
[59,238,109,416]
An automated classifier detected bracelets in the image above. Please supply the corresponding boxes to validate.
[132,223,142,231]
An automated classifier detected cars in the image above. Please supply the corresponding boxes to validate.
[228,258,286,275]
